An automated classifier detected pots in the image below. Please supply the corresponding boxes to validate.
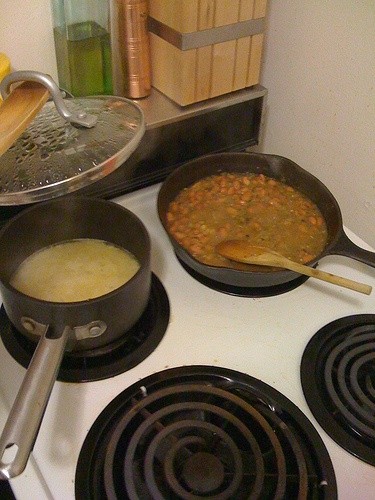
[0,195,151,481]
[158,151,375,287]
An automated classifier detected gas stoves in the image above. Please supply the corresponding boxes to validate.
[0,182,375,500]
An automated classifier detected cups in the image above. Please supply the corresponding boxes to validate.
[51,0,110,97]
[112,0,152,98]
